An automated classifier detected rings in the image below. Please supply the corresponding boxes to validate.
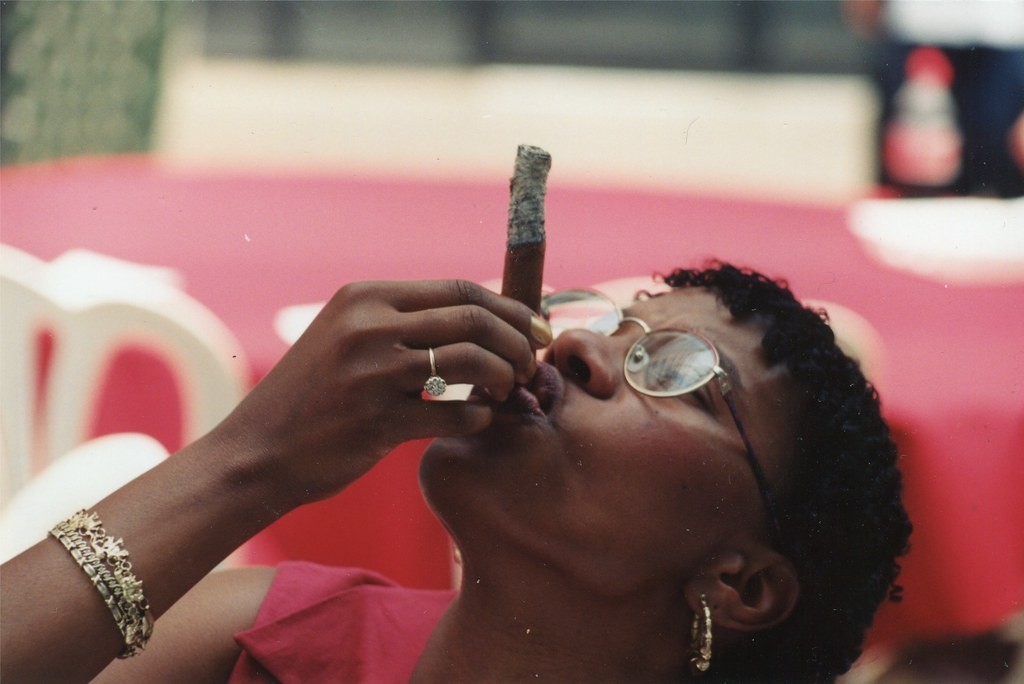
[423,346,447,396]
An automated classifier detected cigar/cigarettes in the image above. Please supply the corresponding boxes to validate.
[502,146,551,387]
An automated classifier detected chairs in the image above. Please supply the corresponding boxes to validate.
[1,245,252,579]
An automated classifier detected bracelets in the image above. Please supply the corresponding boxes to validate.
[48,508,154,660]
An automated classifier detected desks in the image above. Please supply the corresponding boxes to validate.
[2,150,1024,650]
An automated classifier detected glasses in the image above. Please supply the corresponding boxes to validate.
[540,287,778,548]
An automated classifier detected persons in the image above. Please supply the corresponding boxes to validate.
[0,258,912,684]
